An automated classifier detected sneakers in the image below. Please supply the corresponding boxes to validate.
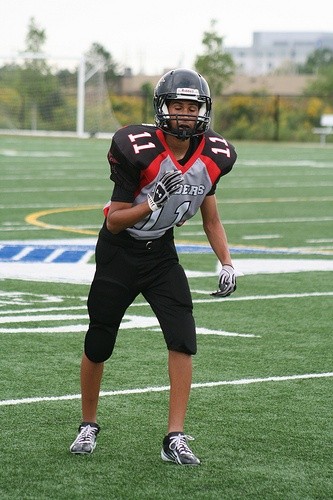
[70,422,101,454]
[161,432,201,466]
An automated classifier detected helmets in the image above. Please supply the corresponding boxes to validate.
[153,69,212,140]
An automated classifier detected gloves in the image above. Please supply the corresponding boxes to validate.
[146,169,184,211]
[210,264,237,297]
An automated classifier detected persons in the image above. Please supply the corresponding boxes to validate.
[69,68,239,467]
